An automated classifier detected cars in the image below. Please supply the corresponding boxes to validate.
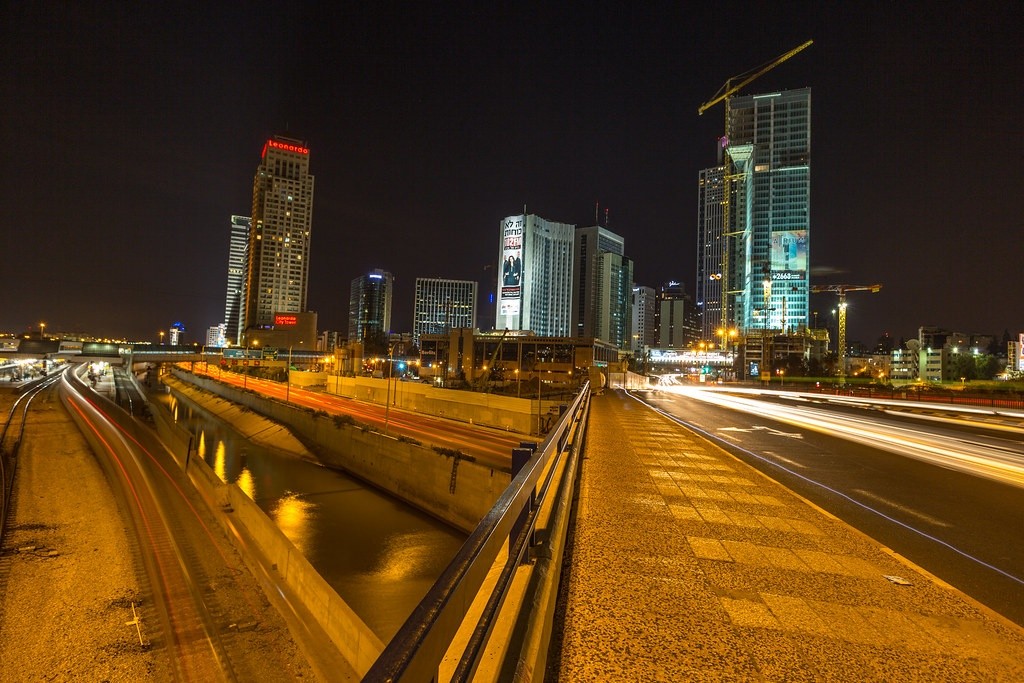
[663,350,678,357]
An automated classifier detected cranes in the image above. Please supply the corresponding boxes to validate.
[698,39,813,353]
[792,284,882,378]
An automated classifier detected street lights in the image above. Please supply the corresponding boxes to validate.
[219,342,230,380]
[385,339,408,435]
[719,331,728,382]
[754,308,776,370]
[529,352,541,435]
[633,335,639,351]
[400,364,403,407]
[41,324,44,340]
[731,331,735,371]
[287,341,304,401]
[408,362,410,371]
[700,344,707,363]
[245,341,257,388]
[160,332,163,342]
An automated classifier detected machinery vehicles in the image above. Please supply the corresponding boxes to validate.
[473,328,511,387]
